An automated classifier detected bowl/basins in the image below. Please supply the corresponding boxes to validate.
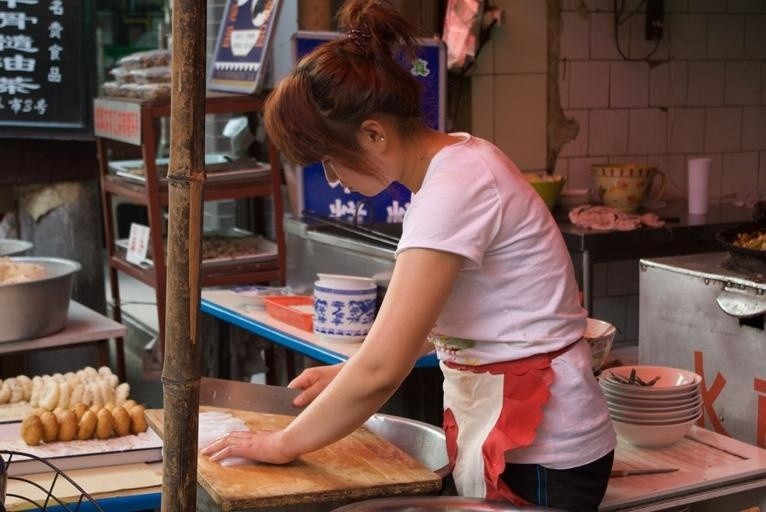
[598,366,706,450]
[0,238,34,254]
[0,255,82,343]
[531,178,566,212]
[364,412,450,479]
[585,317,618,374]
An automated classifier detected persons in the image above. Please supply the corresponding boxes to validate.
[200,0,617,511]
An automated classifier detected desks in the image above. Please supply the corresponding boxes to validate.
[595,424,766,512]
[200,285,444,421]
[0,297,127,381]
[550,201,766,344]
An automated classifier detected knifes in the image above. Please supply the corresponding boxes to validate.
[199,375,311,416]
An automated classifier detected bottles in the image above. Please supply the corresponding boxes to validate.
[312,274,378,343]
[688,158,712,216]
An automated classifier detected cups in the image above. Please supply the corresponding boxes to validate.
[589,163,666,211]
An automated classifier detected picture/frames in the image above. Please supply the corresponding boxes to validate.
[209,0,282,95]
[0,0,98,142]
[291,30,447,266]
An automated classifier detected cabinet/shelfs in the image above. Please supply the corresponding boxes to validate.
[92,84,285,409]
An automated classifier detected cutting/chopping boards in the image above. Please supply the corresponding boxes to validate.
[143,407,444,511]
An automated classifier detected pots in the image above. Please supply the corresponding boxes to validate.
[716,223,766,274]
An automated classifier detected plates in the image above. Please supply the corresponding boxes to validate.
[105,153,270,184]
[114,225,279,265]
